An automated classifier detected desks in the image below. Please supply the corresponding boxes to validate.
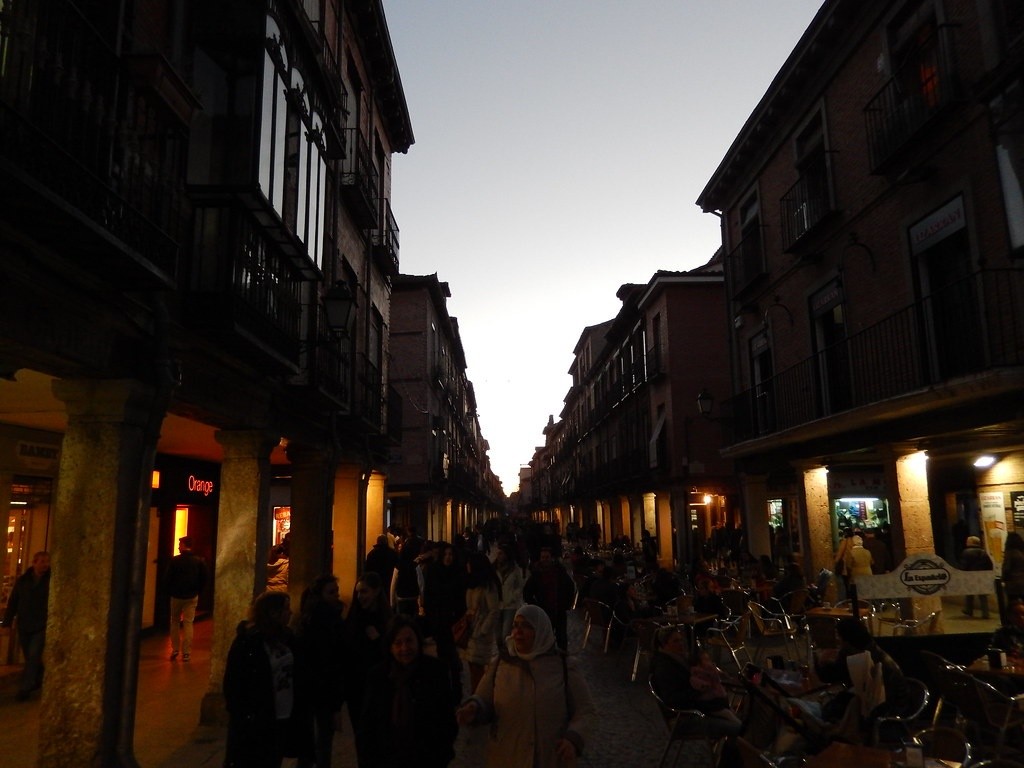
[807,605,867,637]
[969,653,1024,683]
[656,611,719,644]
[782,672,830,700]
[724,586,771,605]
[804,741,963,768]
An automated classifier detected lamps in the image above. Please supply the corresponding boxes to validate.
[298,281,360,357]
[837,231,878,277]
[761,304,795,335]
[696,387,743,433]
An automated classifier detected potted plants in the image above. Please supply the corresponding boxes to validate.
[132,45,206,128]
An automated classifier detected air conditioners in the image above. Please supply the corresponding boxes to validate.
[794,198,821,239]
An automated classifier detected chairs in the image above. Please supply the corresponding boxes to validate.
[576,571,1024,768]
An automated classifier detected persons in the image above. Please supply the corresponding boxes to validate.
[221,517,1024,768]
[2,552,51,699]
[168,537,207,663]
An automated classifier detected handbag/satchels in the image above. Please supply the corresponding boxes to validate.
[452,613,473,644]
[834,559,844,577]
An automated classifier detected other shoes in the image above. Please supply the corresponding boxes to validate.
[184,655,190,661]
[962,608,972,617]
[170,651,179,660]
[15,689,30,703]
[982,614,990,619]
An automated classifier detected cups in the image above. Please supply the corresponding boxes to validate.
[824,602,830,608]
[800,665,809,679]
